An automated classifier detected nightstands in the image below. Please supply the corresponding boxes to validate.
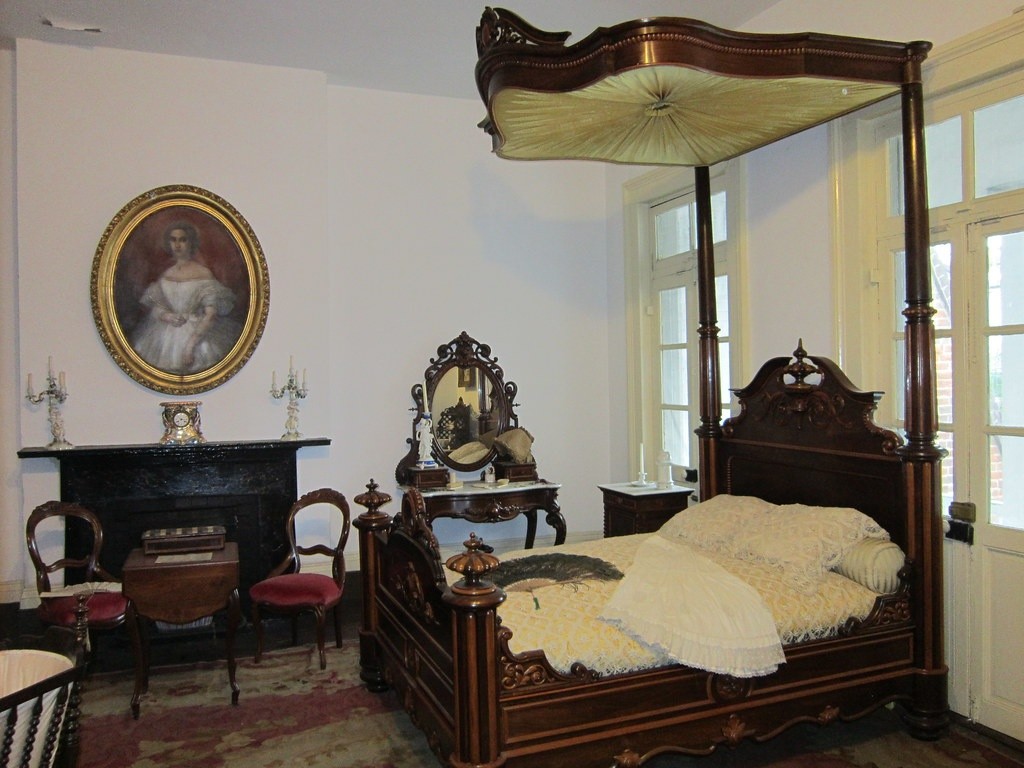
[597,482,696,539]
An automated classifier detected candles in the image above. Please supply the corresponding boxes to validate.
[422,378,429,413]
[273,371,277,385]
[303,369,308,385]
[48,355,54,377]
[290,355,294,376]
[639,442,645,474]
[59,372,65,387]
[28,373,33,389]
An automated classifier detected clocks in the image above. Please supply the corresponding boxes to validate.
[158,400,206,445]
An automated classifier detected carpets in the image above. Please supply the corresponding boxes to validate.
[75,635,1024,768]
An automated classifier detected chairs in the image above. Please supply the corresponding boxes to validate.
[7,626,85,668]
[0,664,86,768]
[248,488,350,669]
[26,501,139,674]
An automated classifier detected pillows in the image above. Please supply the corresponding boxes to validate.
[830,537,906,596]
[656,493,779,556]
[736,503,890,595]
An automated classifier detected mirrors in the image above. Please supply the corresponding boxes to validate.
[430,365,501,466]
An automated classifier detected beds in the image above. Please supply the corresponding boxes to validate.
[350,4,949,768]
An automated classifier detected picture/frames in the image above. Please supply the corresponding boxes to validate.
[90,184,271,396]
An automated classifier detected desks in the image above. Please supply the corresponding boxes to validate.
[118,541,242,723]
[0,649,74,699]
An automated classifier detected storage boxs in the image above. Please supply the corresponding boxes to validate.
[491,460,538,481]
[406,467,450,489]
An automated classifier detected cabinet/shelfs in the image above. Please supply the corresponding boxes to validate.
[15,437,334,661]
